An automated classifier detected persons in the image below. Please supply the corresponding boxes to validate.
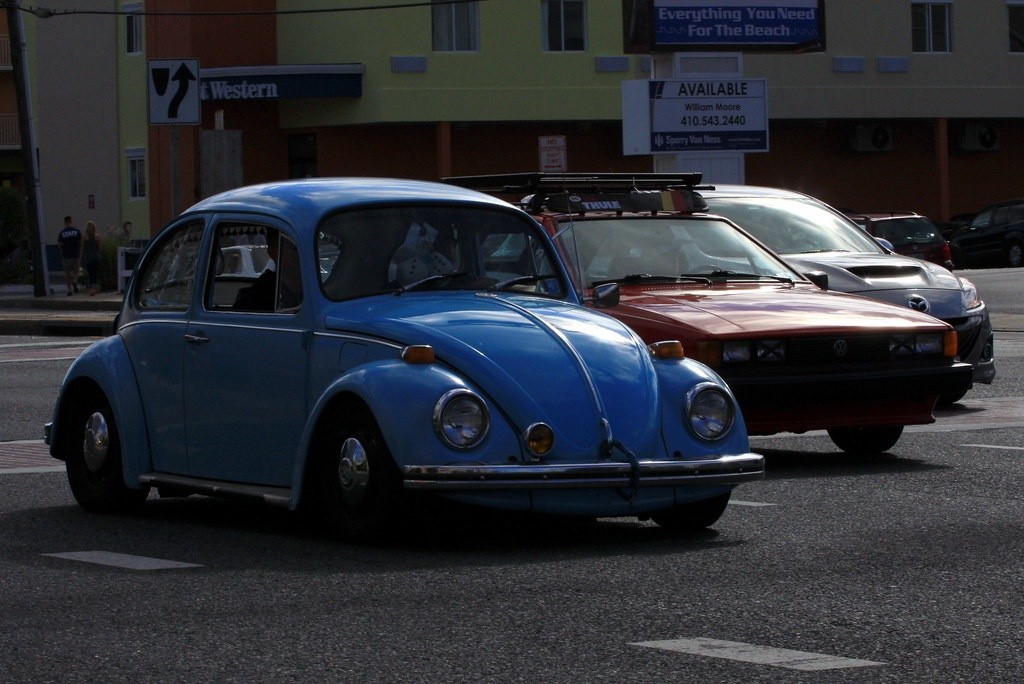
[233,228,302,314]
[57,216,135,297]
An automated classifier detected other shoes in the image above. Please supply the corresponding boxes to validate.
[71,281,79,294]
[67,292,73,296]
[89,289,96,295]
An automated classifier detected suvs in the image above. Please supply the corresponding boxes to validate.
[843,208,956,274]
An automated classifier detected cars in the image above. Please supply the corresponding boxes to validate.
[582,183,1000,416]
[39,178,768,560]
[478,181,974,463]
[934,197,1024,268]
